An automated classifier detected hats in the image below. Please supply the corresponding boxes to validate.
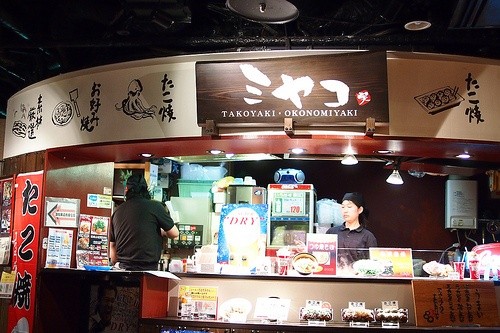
[342,193,366,206]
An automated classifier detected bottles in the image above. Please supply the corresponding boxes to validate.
[183,260,187,273]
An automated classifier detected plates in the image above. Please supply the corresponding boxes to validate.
[84,264,113,271]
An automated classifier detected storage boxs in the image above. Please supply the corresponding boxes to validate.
[177,179,217,198]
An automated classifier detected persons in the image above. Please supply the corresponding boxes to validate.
[109,174,179,271]
[326,192,376,262]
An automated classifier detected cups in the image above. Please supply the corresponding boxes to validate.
[453,261,465,279]
[469,260,481,279]
[235,176,256,185]
[276,250,294,277]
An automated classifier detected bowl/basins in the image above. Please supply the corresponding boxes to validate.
[353,259,385,276]
[292,253,317,275]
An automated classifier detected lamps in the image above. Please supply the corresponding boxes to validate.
[402,0,432,30]
[386,160,403,185]
[341,154,358,165]
